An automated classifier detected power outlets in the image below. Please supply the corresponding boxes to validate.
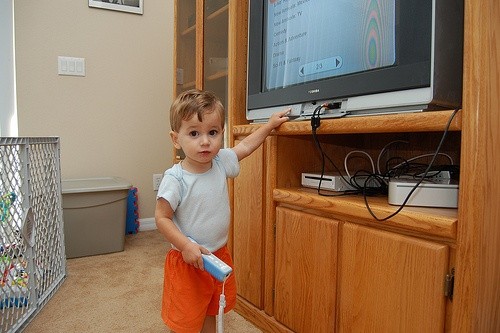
[153,174,162,191]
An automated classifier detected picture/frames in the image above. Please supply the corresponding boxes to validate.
[88,0,144,15]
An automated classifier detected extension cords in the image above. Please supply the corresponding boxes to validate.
[427,171,450,178]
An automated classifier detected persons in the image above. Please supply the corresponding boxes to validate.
[155,88,289,333]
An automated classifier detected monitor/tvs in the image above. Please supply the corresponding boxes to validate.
[246,0,464,124]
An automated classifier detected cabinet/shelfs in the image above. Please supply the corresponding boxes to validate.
[171,0,500,333]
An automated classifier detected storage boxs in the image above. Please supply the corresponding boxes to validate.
[61,176,131,260]
